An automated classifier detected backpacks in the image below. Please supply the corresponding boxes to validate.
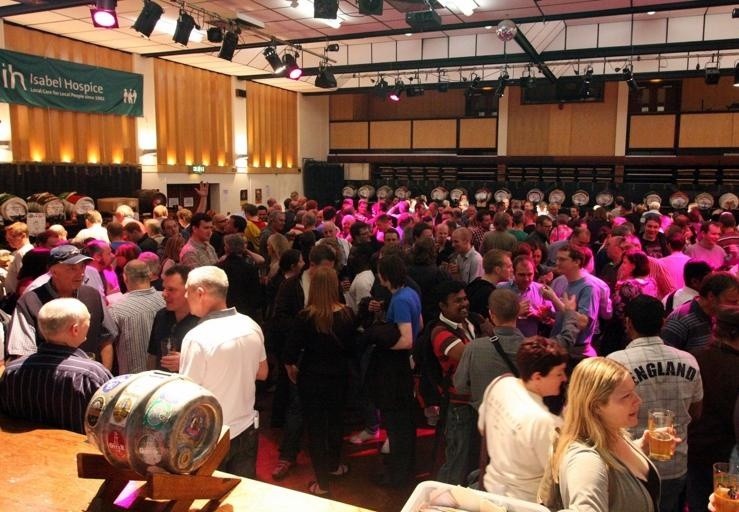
[414,319,464,407]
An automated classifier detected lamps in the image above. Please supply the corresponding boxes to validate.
[77,0,739,105]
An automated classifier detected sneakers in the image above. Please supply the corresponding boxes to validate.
[272,460,292,480]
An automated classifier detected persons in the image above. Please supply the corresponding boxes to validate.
[687,309,738,512]
[608,294,704,512]
[555,356,681,512]
[1,179,739,497]
[476,334,566,502]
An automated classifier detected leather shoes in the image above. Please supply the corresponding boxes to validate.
[328,463,350,479]
[308,480,330,498]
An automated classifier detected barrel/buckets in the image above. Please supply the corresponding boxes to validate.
[84,370,223,479]
[341,186,739,209]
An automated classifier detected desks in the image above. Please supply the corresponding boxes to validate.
[0,412,380,512]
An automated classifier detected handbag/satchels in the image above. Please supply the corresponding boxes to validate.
[436,403,481,466]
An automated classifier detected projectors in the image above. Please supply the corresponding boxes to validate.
[406,12,441,29]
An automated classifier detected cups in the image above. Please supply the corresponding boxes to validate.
[161,338,177,357]
[713,460,739,512]
[648,407,673,460]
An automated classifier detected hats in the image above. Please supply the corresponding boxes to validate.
[47,245,94,267]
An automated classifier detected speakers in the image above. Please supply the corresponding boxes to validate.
[303,160,344,210]
